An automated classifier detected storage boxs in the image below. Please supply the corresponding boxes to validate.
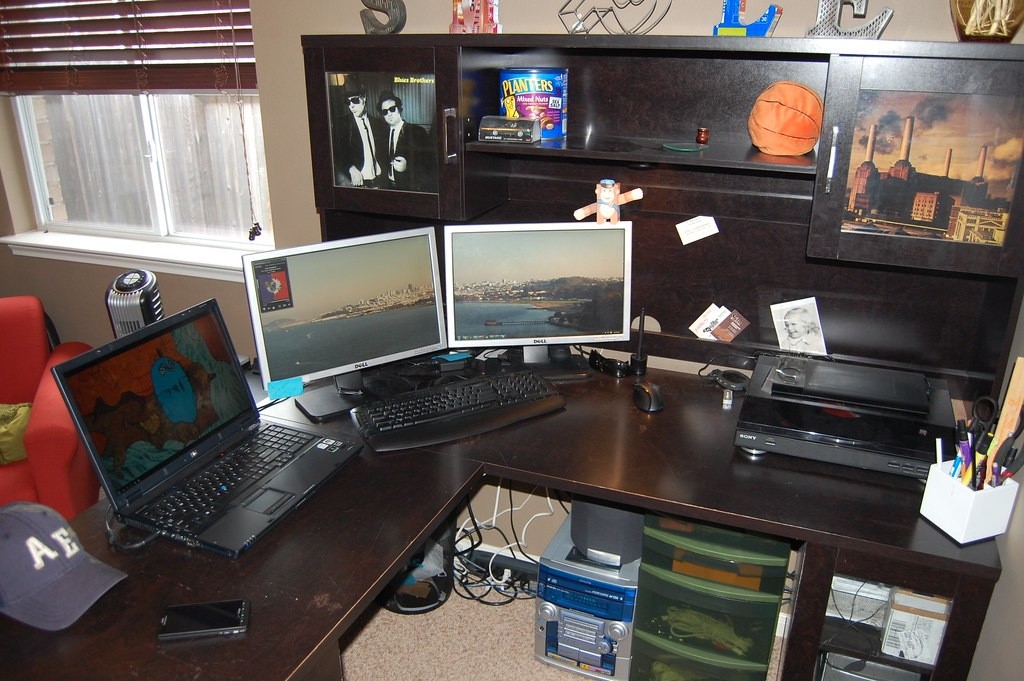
[629,515,792,681]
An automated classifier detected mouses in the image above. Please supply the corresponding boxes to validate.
[633,382,665,412]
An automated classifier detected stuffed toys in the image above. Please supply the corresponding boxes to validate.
[748,82,824,156]
[574,179,644,224]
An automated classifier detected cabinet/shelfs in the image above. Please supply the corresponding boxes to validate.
[298,32,1024,389]
[0,355,1002,681]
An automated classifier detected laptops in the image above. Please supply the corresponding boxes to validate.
[51,298,363,559]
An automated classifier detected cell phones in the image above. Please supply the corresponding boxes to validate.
[158,599,252,641]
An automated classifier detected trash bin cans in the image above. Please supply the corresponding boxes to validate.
[383,517,456,615]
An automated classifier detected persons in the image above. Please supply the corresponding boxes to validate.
[782,307,824,353]
[376,88,437,193]
[333,79,394,190]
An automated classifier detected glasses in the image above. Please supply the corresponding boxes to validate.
[382,106,397,116]
[344,98,359,106]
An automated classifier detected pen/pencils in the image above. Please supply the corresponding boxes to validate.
[935,417,1024,491]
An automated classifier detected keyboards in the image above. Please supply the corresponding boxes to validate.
[350,370,567,452]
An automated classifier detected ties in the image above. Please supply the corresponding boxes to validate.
[389,129,395,176]
[362,118,376,177]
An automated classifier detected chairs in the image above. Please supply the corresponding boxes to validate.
[0,295,101,520]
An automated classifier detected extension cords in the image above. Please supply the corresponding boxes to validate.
[466,550,538,584]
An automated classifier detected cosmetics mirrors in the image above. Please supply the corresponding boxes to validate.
[663,143,709,152]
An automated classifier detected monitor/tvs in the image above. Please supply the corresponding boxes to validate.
[241,226,447,423]
[444,221,633,380]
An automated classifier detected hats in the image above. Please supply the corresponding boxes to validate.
[0,502,128,632]
[376,90,401,112]
[343,75,367,97]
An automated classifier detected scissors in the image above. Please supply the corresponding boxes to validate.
[973,395,1024,434]
